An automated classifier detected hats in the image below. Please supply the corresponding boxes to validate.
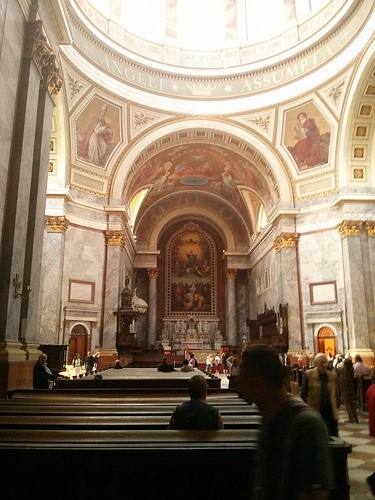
[242,344,291,378]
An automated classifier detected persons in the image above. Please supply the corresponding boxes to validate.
[233,343,337,500]
[363,368,375,500]
[31,353,58,394]
[352,354,372,378]
[299,351,340,439]
[336,354,360,424]
[71,348,344,389]
[165,375,225,432]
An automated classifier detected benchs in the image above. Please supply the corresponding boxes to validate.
[1,365,352,500]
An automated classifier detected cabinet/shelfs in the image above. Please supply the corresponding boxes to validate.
[157,338,222,356]
